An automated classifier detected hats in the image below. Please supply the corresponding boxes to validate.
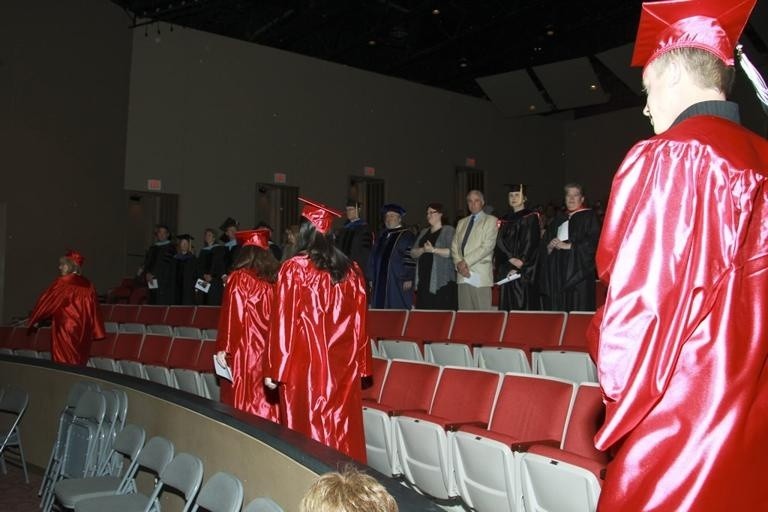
[298,197,343,233]
[505,184,529,192]
[177,234,194,241]
[234,229,271,250]
[253,221,273,232]
[156,224,171,233]
[64,249,87,267]
[380,203,406,216]
[630,0,757,74]
[219,217,240,231]
[346,198,362,208]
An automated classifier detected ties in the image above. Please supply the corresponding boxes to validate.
[461,216,478,257]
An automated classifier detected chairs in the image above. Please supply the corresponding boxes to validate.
[174,306,223,338]
[116,303,171,333]
[450,373,577,512]
[148,305,197,337]
[114,330,145,382]
[35,326,54,363]
[142,452,204,512]
[473,307,567,375]
[140,332,172,388]
[76,435,173,512]
[423,310,508,367]
[0,326,13,354]
[167,338,201,397]
[512,379,615,511]
[390,365,503,512]
[202,326,217,335]
[378,306,454,365]
[359,352,392,404]
[191,472,245,511]
[52,423,147,512]
[92,330,121,373]
[0,383,32,485]
[12,325,39,361]
[37,379,130,511]
[537,309,599,386]
[198,339,222,401]
[97,301,115,324]
[364,361,444,483]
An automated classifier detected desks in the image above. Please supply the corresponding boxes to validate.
[0,353,447,511]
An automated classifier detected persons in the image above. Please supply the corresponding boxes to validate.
[494,183,540,312]
[533,197,563,230]
[409,201,455,310]
[137,225,175,306]
[214,229,277,421]
[160,235,196,304]
[587,197,608,220]
[589,0,767,512]
[451,191,497,311]
[195,229,217,303]
[368,204,417,309]
[261,195,375,464]
[542,183,599,313]
[255,221,281,260]
[332,196,372,312]
[25,252,105,366]
[219,218,240,282]
